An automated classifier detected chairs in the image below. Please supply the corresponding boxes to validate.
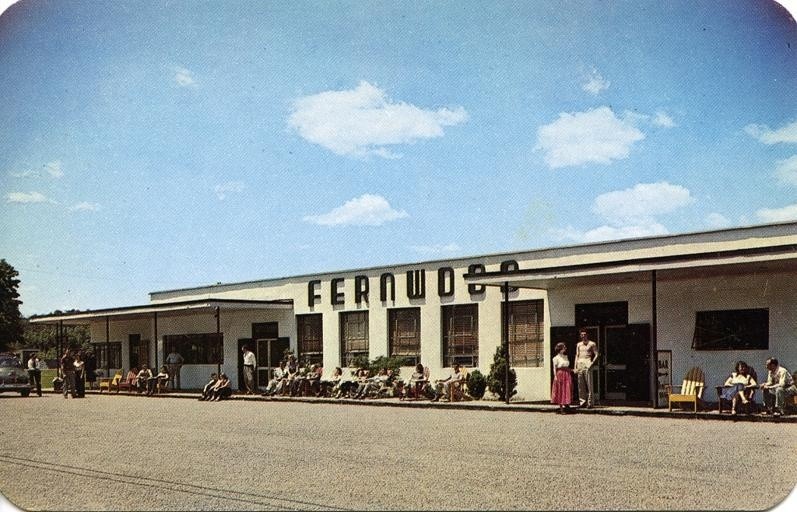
[666,367,707,413]
[267,365,470,404]
[95,366,174,396]
[785,372,797,412]
[715,365,759,414]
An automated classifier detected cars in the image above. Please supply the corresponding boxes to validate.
[0,356,32,398]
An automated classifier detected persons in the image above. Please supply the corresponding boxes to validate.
[126,346,185,396]
[198,370,217,402]
[61,348,76,397]
[26,351,44,397]
[72,351,87,397]
[240,344,258,396]
[211,372,232,401]
[572,328,598,409]
[550,341,575,414]
[260,352,465,403]
[721,360,758,415]
[758,357,796,418]
